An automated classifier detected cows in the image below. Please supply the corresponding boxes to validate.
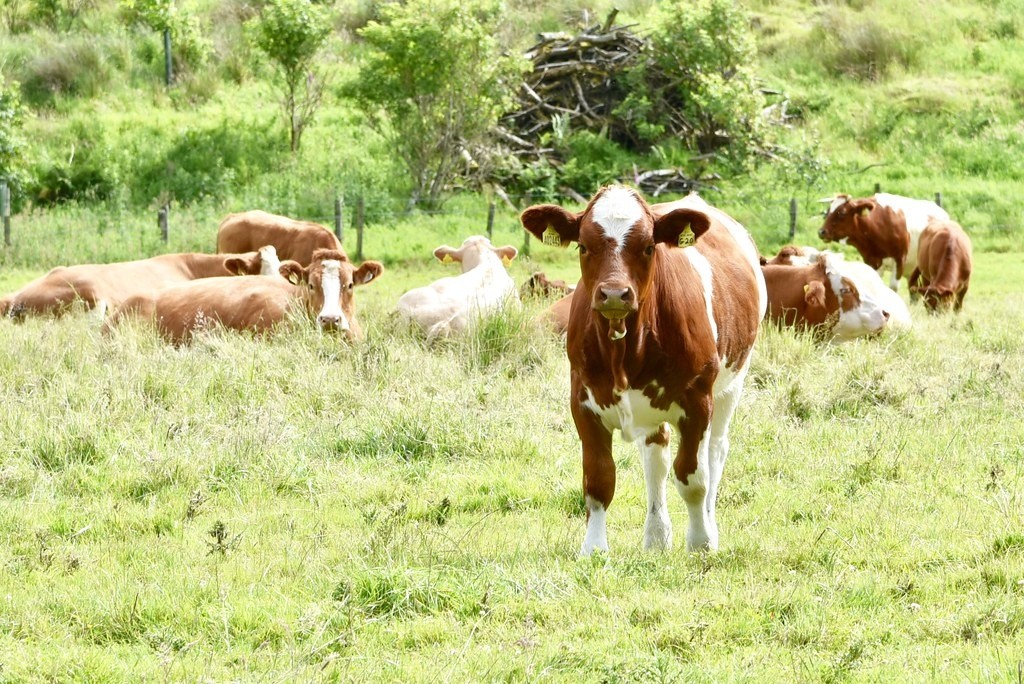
[390,234,522,342]
[816,192,972,317]
[520,274,574,297]
[533,255,890,352]
[101,248,385,350]
[765,246,810,265]
[0,244,280,323]
[520,183,768,561]
[216,209,347,269]
